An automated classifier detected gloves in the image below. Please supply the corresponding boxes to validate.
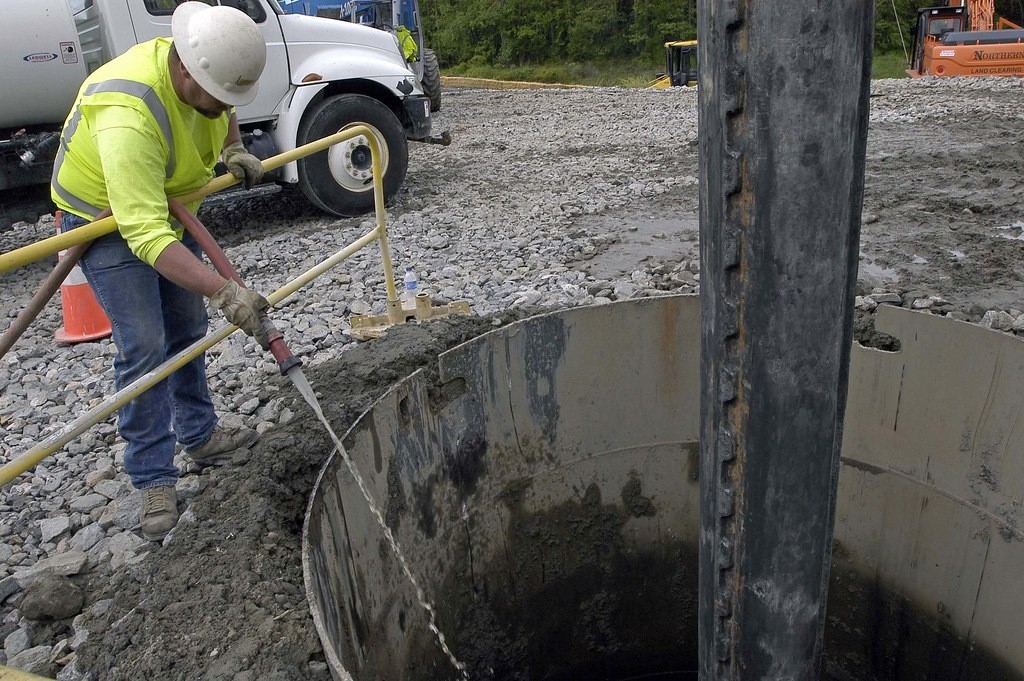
[209,277,271,351]
[222,142,264,191]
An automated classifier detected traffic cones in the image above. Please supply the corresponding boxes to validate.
[53,209,113,344]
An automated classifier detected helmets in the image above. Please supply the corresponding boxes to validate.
[171,1,266,106]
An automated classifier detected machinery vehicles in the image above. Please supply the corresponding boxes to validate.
[903,0,1024,80]
[1,0,453,219]
[648,39,698,89]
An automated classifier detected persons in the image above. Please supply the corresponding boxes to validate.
[51,2,271,541]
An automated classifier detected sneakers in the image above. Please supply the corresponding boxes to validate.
[190,421,259,462]
[140,486,179,541]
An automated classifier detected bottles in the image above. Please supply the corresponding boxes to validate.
[404,266,417,309]
